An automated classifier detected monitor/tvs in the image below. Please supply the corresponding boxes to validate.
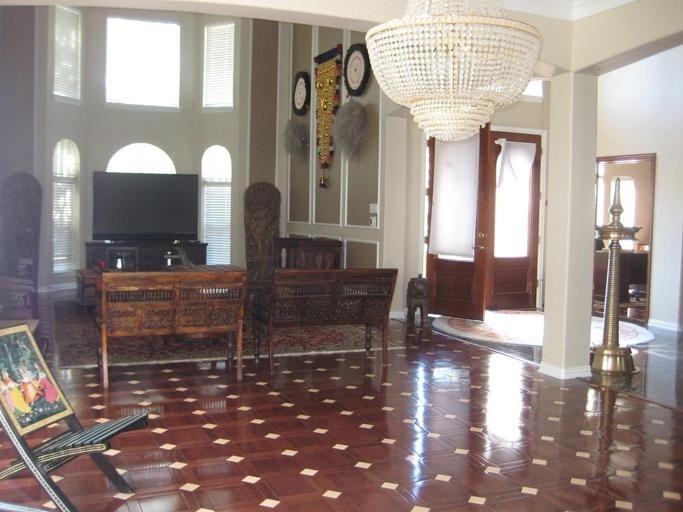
[92,170,198,247]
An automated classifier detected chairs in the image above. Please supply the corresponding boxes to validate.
[0,324,149,512]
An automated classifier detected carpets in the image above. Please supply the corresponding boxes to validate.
[53,299,405,370]
[431,309,655,348]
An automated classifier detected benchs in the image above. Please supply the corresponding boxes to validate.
[94,271,247,386]
[253,268,398,365]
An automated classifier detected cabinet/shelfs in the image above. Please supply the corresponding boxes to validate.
[86,241,207,270]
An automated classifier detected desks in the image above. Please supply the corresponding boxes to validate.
[272,237,343,272]
[74,264,253,321]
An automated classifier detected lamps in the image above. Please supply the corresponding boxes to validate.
[365,0,543,142]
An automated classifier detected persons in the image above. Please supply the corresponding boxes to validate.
[0,361,58,415]
